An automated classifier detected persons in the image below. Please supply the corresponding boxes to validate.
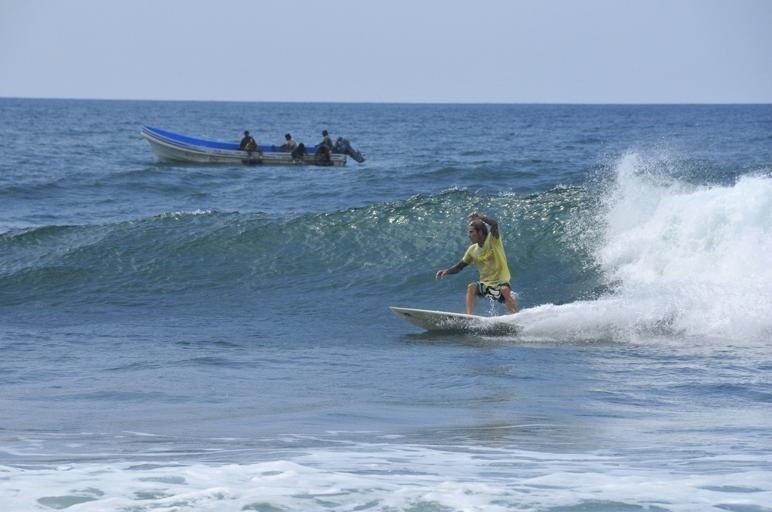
[334,136,347,153]
[241,130,257,151]
[434,210,519,317]
[316,141,330,162]
[314,128,334,152]
[244,138,264,159]
[280,133,297,152]
[290,143,308,164]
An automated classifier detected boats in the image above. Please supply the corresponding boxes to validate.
[139,125,365,167]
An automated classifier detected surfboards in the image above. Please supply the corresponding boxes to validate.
[390,306,523,335]
